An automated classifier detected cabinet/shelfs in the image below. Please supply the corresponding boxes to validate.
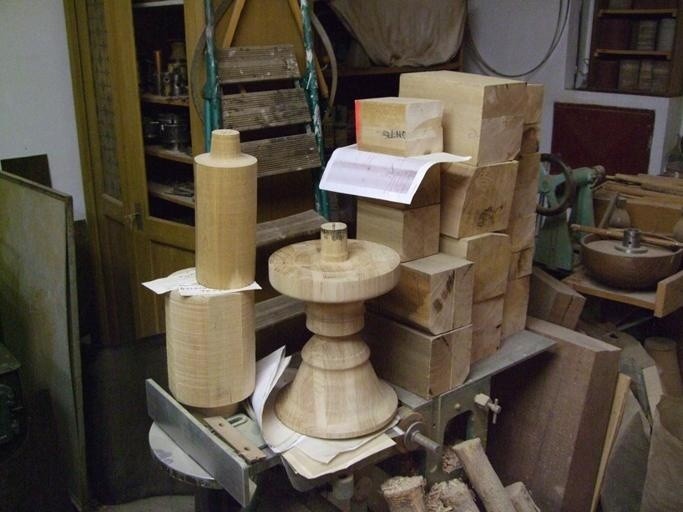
[586,0,683,97]
[60,2,316,359]
